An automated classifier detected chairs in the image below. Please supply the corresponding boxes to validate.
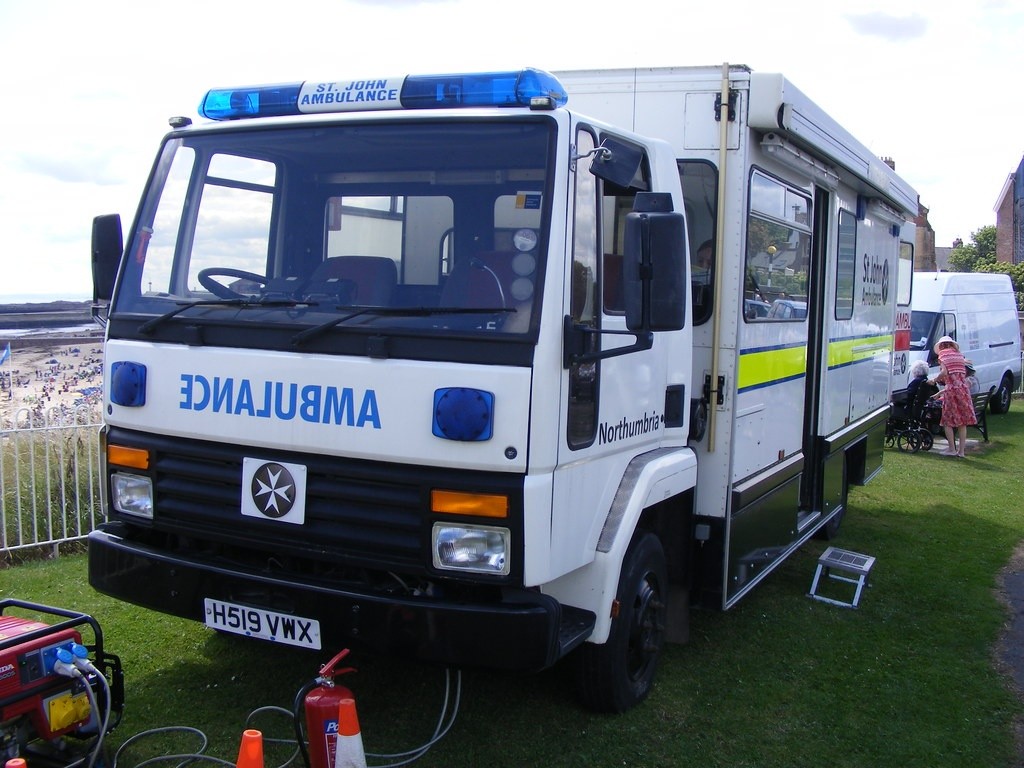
[440,253,585,320]
[304,256,396,311]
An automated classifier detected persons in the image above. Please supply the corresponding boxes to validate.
[927,359,980,418]
[886,360,939,435]
[664,239,714,315]
[0,347,103,406]
[926,336,977,458]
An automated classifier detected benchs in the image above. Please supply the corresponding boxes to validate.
[927,384,996,450]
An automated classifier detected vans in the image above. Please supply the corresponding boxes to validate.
[907,271,1021,414]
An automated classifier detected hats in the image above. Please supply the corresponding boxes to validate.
[934,336,959,355]
[963,359,976,372]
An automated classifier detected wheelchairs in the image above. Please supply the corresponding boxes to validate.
[884,382,941,455]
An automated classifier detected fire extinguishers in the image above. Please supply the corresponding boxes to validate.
[292,646,355,768]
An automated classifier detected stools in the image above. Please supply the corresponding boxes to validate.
[807,545,877,610]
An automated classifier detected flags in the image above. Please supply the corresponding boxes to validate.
[0,344,10,364]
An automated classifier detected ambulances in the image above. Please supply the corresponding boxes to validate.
[87,57,919,713]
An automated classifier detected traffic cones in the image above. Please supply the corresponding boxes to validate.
[334,699,369,768]
[235,728,264,768]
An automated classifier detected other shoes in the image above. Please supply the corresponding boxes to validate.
[956,450,965,458]
[939,449,957,456]
